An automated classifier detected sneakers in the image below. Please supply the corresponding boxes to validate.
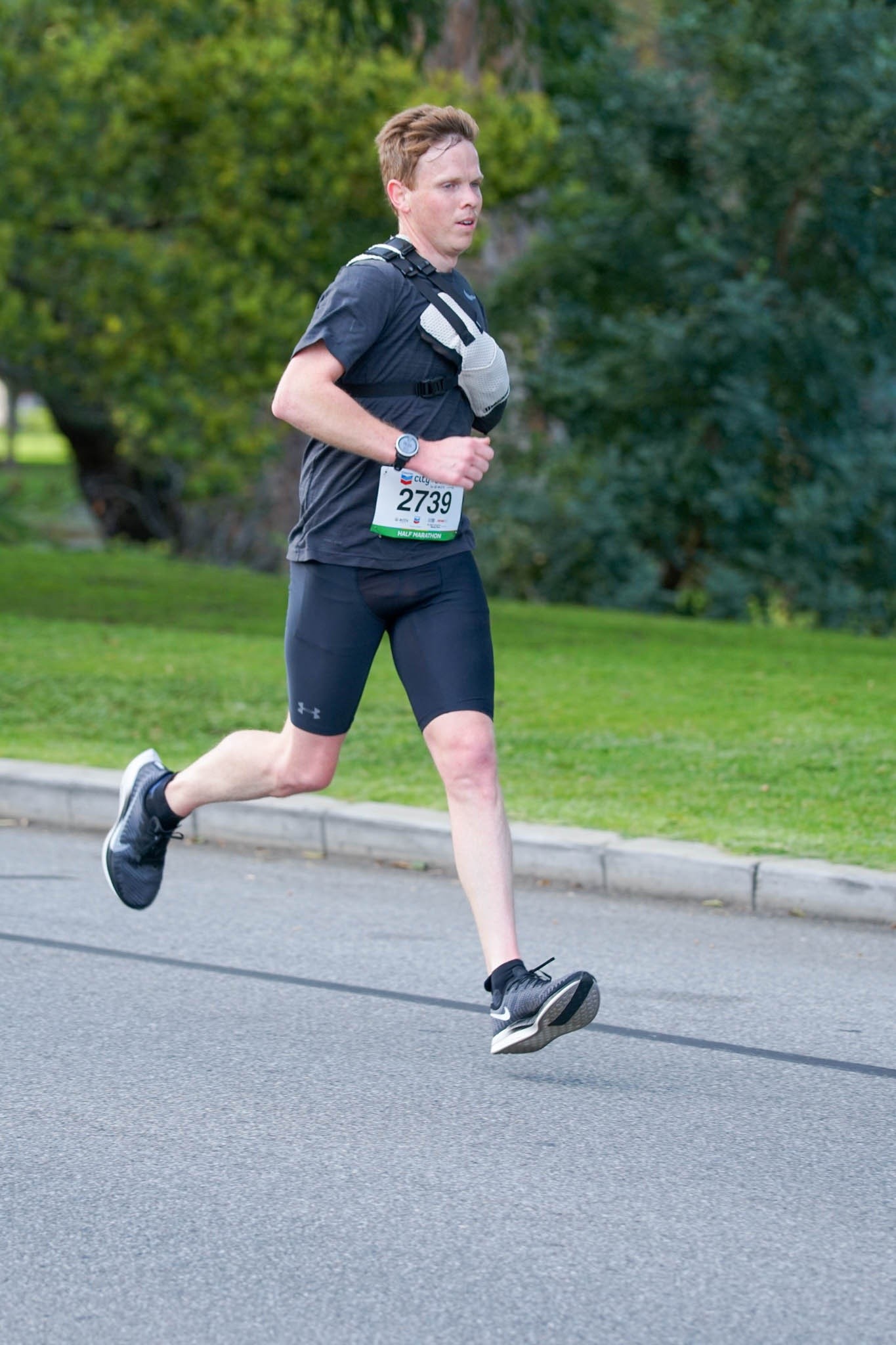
[488,956,600,1055]
[101,748,184,910]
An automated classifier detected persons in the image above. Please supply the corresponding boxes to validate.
[103,103,599,1053]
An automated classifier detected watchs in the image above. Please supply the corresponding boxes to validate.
[393,432,420,471]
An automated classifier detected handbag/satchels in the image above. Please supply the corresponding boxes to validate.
[365,237,511,435]
